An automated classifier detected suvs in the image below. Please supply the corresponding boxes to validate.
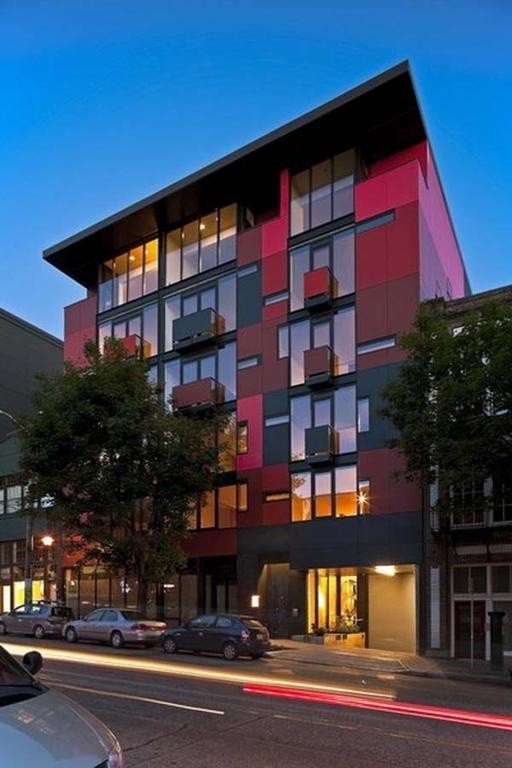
[0,599,74,639]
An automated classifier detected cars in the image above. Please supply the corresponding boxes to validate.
[159,612,270,662]
[61,608,167,649]
[0,645,123,768]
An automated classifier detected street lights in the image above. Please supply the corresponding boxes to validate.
[40,536,54,600]
